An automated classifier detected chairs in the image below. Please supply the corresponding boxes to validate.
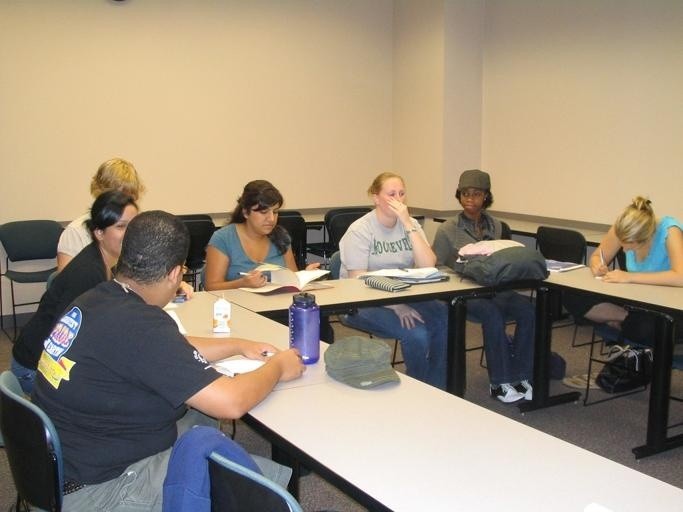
[277,211,307,270]
[0,220,65,340]
[325,207,403,365]
[174,215,216,290]
[0,370,64,512]
[207,451,313,512]
[536,226,587,273]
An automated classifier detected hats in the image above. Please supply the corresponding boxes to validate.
[323,335,399,390]
[457,169,491,192]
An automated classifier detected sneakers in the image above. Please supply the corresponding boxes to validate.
[489,382,521,404]
[515,380,534,401]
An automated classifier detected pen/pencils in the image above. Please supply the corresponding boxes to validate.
[599,248,605,265]
[236,271,250,276]
[261,351,310,361]
[398,267,408,272]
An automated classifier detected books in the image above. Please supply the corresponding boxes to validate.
[238,261,334,294]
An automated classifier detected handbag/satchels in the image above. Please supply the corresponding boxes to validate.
[598,348,656,393]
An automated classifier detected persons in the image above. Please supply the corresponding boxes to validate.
[9,190,139,401]
[432,171,542,403]
[31,210,307,512]
[56,159,194,301]
[559,198,683,349]
[200,180,334,346]
[337,173,446,391]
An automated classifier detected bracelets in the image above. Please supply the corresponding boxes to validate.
[406,227,417,235]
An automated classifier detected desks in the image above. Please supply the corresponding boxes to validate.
[166,290,683,512]
[546,262,683,460]
[433,210,626,273]
[207,206,430,241]
[207,263,582,411]
